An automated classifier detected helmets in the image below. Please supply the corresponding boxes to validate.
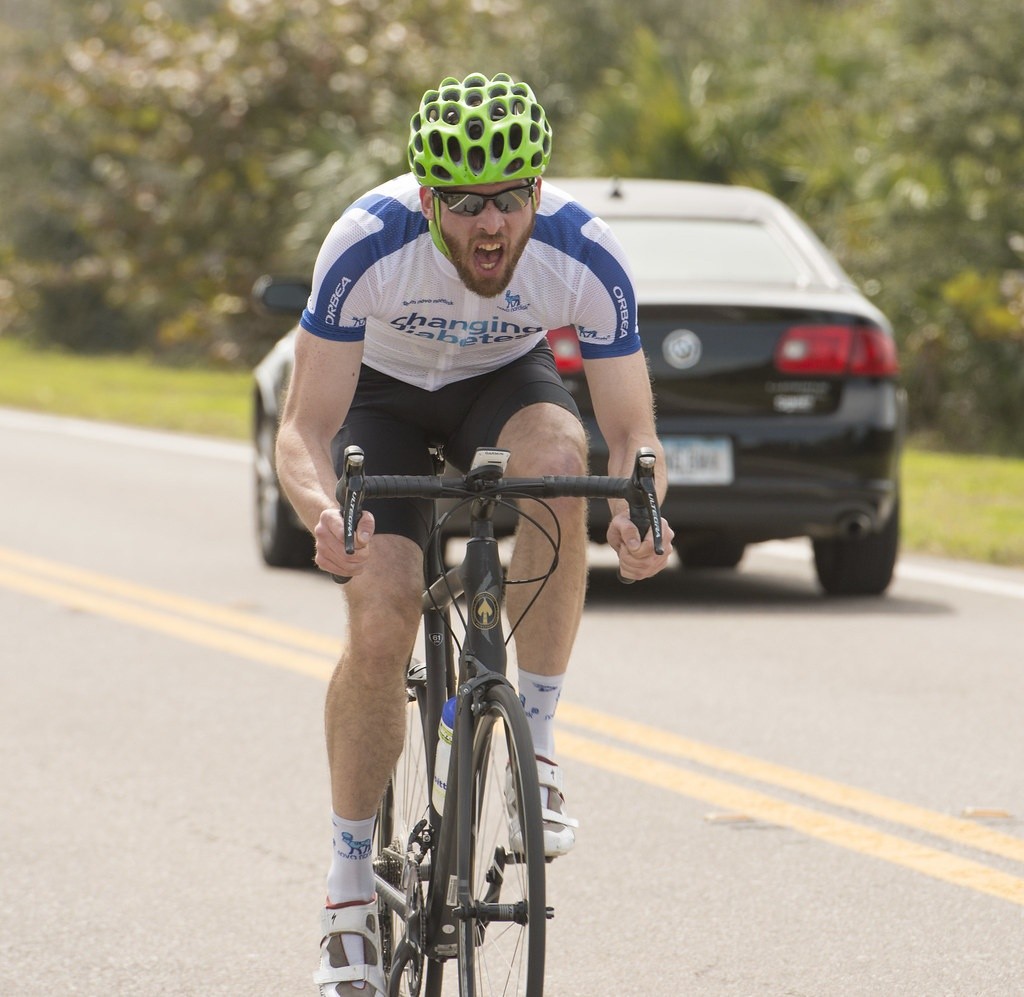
[407,72,554,187]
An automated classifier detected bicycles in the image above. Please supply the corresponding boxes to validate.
[333,446,668,997]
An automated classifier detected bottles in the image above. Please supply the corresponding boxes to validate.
[431,696,457,818]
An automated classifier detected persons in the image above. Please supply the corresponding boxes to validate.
[276,73,671,997]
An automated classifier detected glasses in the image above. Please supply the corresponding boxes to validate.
[430,177,537,217]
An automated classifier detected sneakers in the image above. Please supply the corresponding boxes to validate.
[505,754,577,857]
[314,892,387,997]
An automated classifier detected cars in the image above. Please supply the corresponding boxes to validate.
[248,171,911,600]
